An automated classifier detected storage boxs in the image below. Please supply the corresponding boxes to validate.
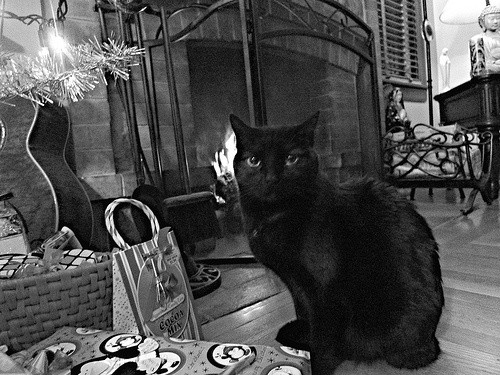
[7,327,312,375]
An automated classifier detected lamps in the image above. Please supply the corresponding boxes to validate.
[440,0,500,25]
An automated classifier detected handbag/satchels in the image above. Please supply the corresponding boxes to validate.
[103,197,205,341]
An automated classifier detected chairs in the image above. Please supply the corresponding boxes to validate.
[384,83,494,214]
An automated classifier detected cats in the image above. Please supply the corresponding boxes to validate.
[228,113,447,375]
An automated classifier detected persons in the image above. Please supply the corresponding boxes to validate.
[387,88,416,139]
[212,151,231,186]
[440,48,451,88]
[469,4,500,73]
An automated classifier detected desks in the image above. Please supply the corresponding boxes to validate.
[433,73,500,200]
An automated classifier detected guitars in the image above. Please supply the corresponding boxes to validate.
[0,56,94,252]
